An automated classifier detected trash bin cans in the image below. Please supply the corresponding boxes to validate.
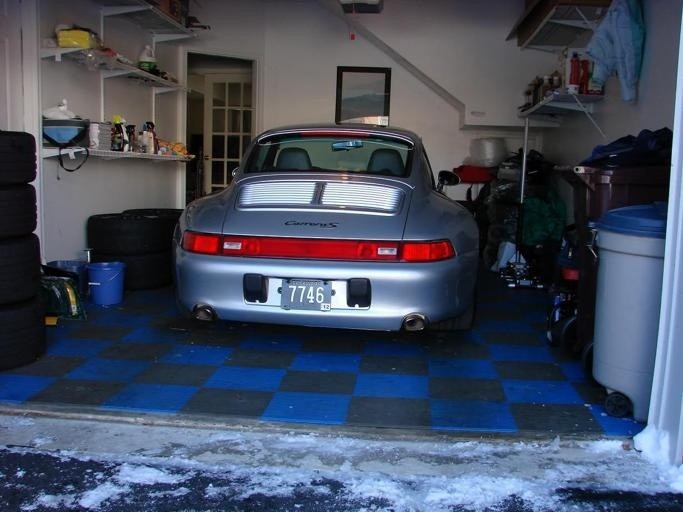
[586,202,667,422]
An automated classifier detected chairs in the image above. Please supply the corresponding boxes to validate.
[276,147,312,171]
[367,148,404,176]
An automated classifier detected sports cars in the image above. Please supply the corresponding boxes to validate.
[174,120,482,337]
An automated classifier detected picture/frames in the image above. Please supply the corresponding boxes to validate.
[335,66,392,127]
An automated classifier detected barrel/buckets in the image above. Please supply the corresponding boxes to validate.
[85,261,126,306]
[46,259,86,306]
[139,44,158,73]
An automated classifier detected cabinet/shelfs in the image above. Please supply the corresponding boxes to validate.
[517,0,611,139]
[41,5,198,161]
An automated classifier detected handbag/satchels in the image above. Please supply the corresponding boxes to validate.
[40,274,88,322]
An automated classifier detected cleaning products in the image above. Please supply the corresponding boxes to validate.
[138,121,160,154]
[111,113,130,152]
